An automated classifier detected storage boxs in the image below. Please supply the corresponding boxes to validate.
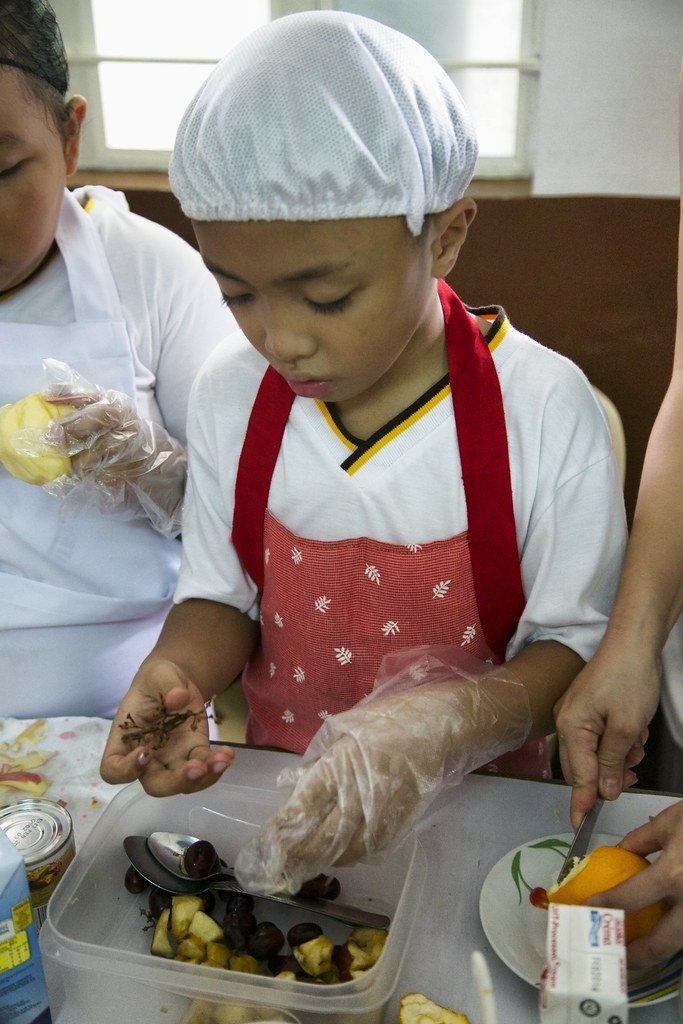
[38,776,431,1023]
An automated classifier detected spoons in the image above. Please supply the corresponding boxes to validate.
[146,830,241,882]
[122,834,393,931]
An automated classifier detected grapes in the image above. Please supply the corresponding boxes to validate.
[124,839,344,969]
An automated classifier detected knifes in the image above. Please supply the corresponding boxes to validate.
[557,793,604,886]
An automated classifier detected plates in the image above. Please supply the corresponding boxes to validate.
[480,832,683,1008]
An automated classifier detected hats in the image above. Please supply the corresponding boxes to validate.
[169,11,478,237]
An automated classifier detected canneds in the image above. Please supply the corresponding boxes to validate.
[0,797,76,936]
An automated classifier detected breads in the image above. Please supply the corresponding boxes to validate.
[1,391,82,485]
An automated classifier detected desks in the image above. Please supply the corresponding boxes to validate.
[51,739,682,1024]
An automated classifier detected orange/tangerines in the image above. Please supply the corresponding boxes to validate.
[544,843,665,949]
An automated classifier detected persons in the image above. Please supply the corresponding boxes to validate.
[101,10,627,862]
[0,0,239,741]
[552,250,683,971]
